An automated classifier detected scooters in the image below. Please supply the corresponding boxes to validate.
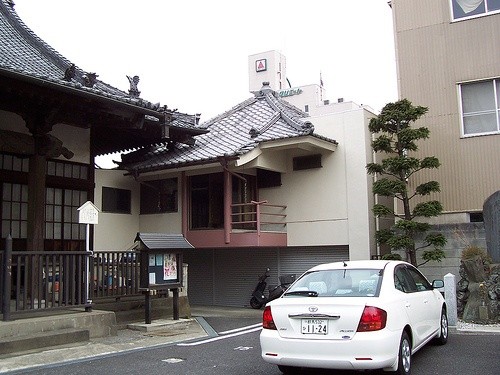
[250,265,296,309]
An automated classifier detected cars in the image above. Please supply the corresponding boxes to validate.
[259,259,449,375]
